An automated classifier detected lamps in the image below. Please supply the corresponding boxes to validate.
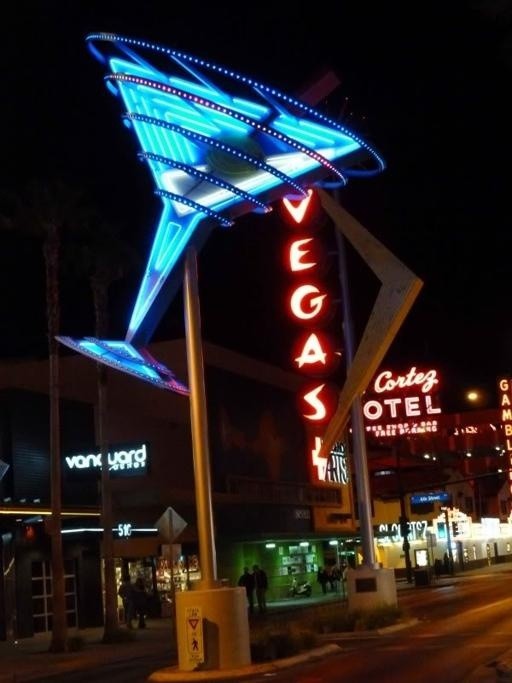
[266,542,275,549]
[329,540,338,546]
[299,542,310,547]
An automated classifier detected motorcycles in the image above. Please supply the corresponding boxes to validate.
[287,574,314,599]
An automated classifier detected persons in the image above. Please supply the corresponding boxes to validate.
[443,552,448,568]
[119,574,148,629]
[134,573,147,627]
[251,564,269,619]
[317,562,353,595]
[237,566,256,614]
